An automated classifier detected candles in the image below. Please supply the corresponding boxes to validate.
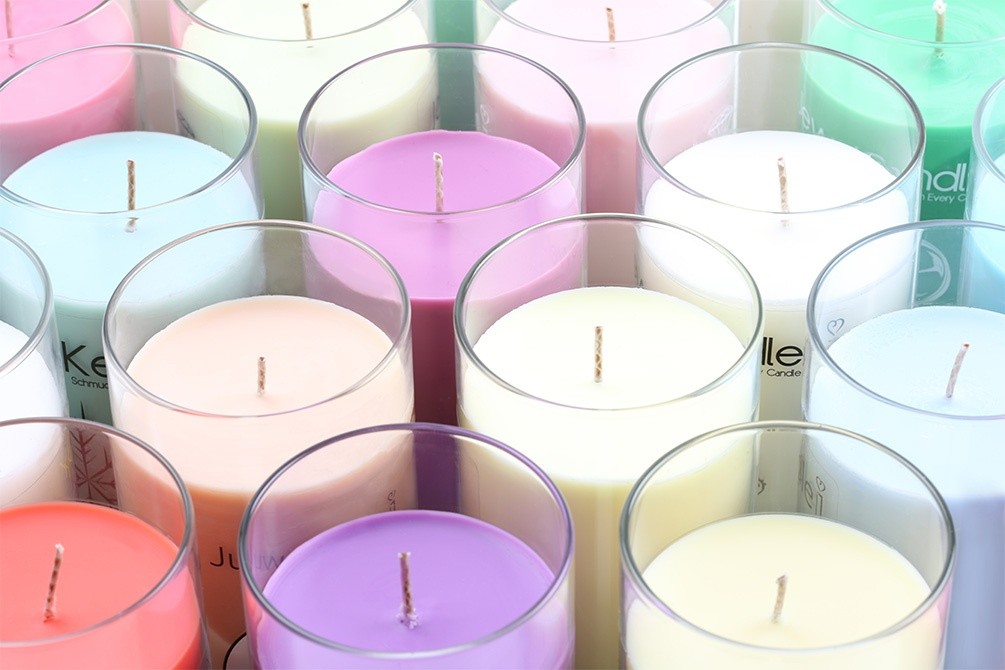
[0,0,1005,670]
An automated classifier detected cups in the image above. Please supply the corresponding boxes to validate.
[0,0,1005,670]
